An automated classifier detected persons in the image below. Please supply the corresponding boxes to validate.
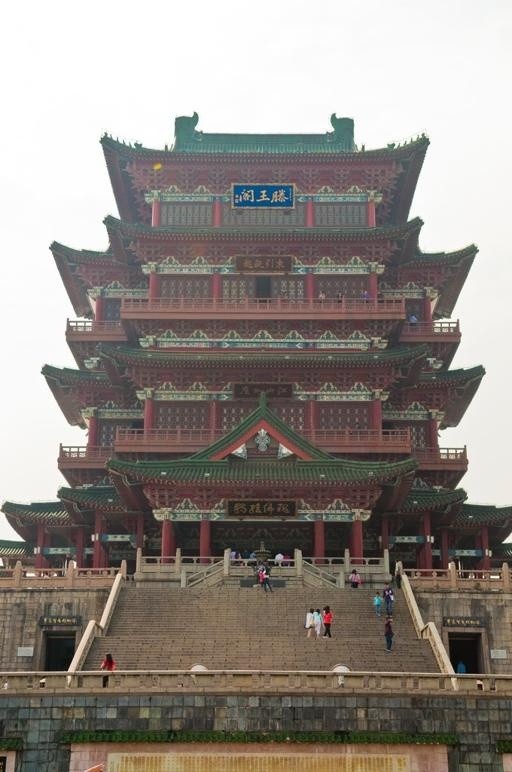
[100,653,115,688]
[457,660,466,673]
[229,548,291,592]
[303,605,334,639]
[349,569,361,588]
[369,592,383,617]
[393,560,402,588]
[382,582,393,616]
[383,615,395,652]
